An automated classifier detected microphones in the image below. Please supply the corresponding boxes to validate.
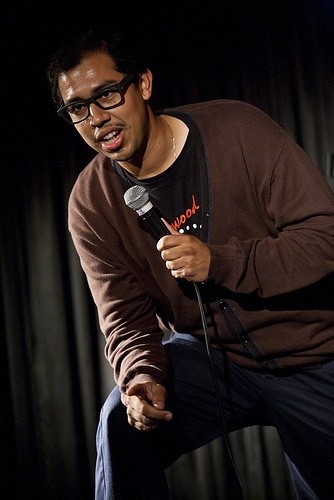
[122,186,172,243]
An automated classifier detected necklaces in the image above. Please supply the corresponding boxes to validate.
[123,119,178,178]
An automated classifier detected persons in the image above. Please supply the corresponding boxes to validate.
[43,29,334,500]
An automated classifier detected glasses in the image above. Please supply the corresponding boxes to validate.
[56,69,136,125]
